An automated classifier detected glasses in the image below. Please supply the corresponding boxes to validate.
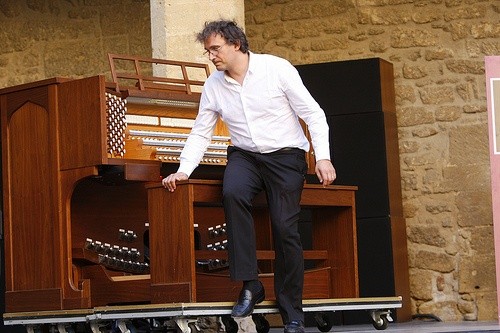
[202,41,229,58]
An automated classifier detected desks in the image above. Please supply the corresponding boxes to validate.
[146,179,359,304]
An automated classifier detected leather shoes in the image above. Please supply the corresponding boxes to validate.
[229,281,267,316]
[283,322,304,333]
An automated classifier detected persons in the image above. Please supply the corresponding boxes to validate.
[162,19,337,333]
